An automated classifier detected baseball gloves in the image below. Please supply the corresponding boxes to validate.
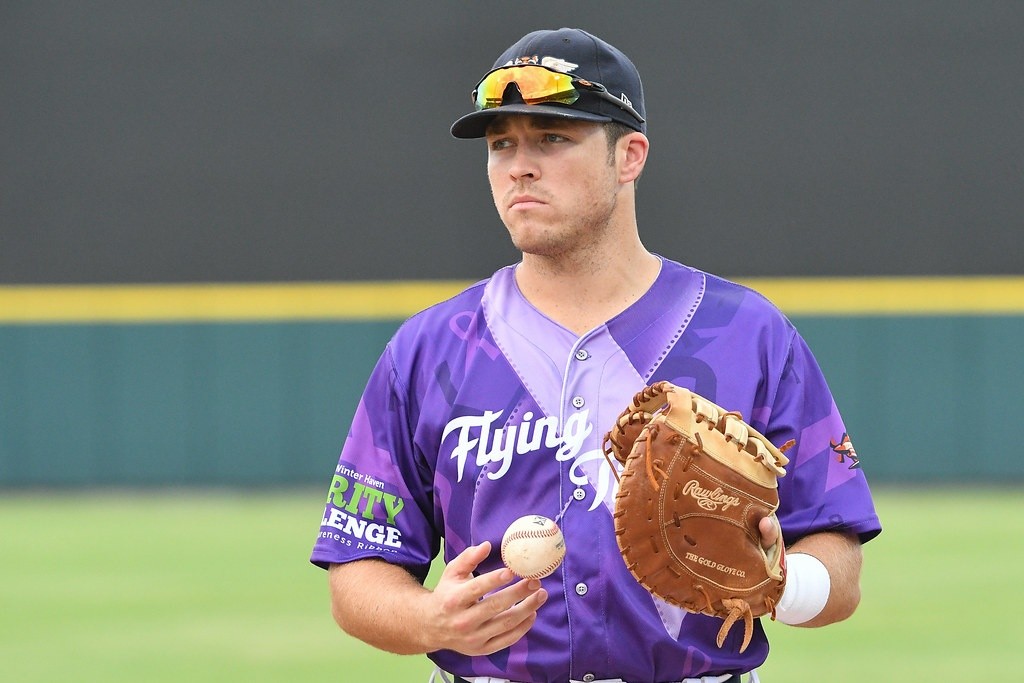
[602,379,789,622]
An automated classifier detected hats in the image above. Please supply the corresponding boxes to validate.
[449,28,646,140]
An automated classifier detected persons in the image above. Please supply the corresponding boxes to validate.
[310,28,882,683]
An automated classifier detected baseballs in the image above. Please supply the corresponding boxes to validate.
[499,513,567,580]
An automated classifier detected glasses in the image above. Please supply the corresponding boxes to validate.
[471,64,645,124]
